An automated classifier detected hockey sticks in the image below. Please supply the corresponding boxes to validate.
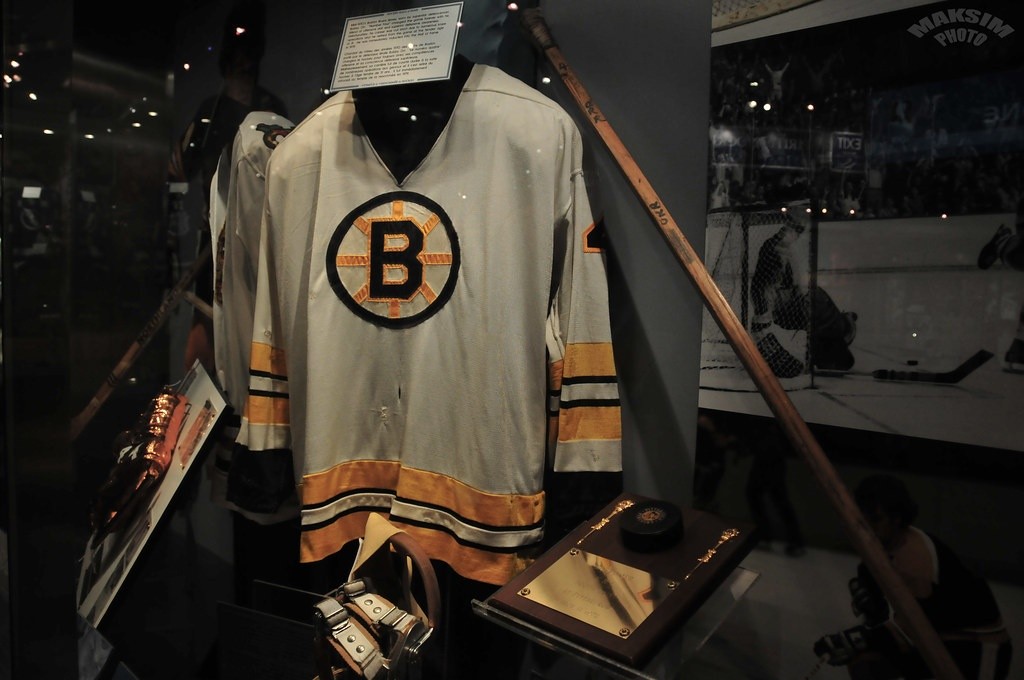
[31,241,212,446]
[813,349,994,385]
[164,211,213,317]
[800,653,830,680]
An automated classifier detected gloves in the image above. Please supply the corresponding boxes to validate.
[847,576,874,611]
[812,621,885,666]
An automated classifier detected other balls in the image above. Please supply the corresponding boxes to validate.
[906,360,918,364]
[619,499,685,551]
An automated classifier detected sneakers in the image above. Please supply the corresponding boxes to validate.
[975,223,1014,270]
[1001,338,1024,371]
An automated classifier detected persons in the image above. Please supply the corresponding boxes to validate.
[978,197,1024,270]
[1003,302,1024,363]
[751,205,857,343]
[180,60,289,503]
[236,52,623,587]
[865,489,938,597]
